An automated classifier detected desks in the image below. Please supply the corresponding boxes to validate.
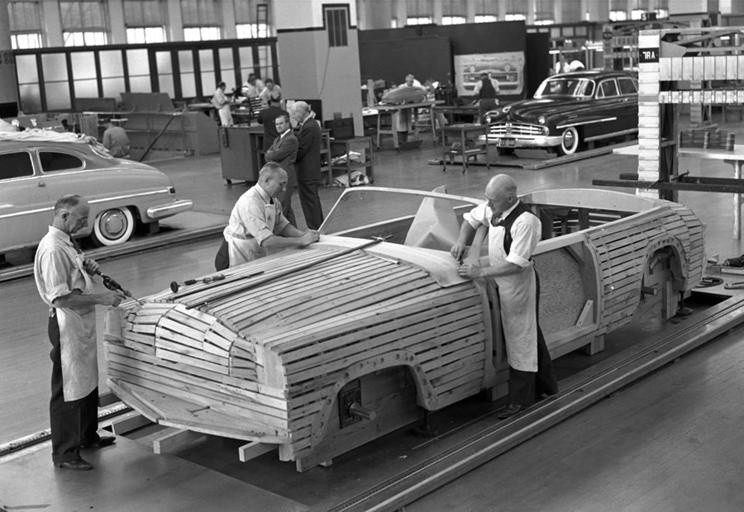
[369,100,445,151]
[188,103,250,126]
[433,107,480,124]
[612,143,744,239]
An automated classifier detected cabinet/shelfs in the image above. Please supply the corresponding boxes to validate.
[439,123,490,173]
[330,137,374,188]
[249,128,333,187]
[82,111,186,157]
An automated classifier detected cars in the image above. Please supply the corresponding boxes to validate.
[89,188,708,476]
[481,66,640,156]
[0,128,194,261]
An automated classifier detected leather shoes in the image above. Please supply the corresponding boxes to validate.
[54,458,93,471]
[80,436,116,449]
[497,401,525,418]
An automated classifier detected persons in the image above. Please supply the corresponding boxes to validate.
[32,192,127,473]
[450,174,559,418]
[289,100,324,231]
[395,72,501,143]
[215,161,320,270]
[100,118,130,158]
[212,73,284,153]
[265,110,299,228]
